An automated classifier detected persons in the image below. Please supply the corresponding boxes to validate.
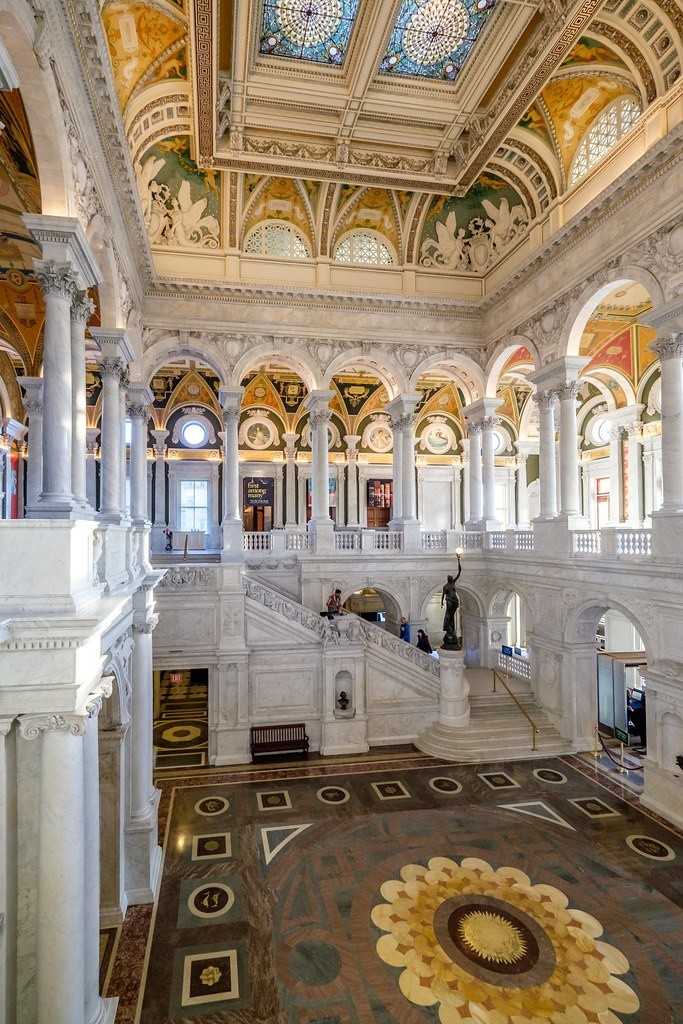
[399,617,410,643]
[338,691,349,706]
[441,565,462,639]
[416,629,432,654]
[326,589,341,613]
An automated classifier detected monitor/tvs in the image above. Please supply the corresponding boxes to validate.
[631,690,643,703]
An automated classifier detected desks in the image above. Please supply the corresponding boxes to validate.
[171,531,206,550]
